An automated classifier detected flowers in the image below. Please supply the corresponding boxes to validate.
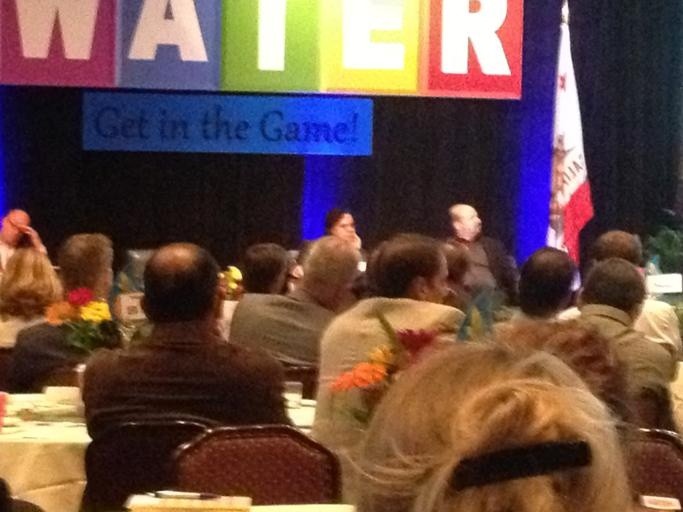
[219,266,243,299]
[45,288,113,359]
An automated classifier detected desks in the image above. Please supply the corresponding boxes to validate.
[0,387,317,512]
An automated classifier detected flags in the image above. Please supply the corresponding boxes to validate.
[542,0,595,293]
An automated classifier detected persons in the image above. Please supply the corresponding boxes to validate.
[0,204,683,512]
